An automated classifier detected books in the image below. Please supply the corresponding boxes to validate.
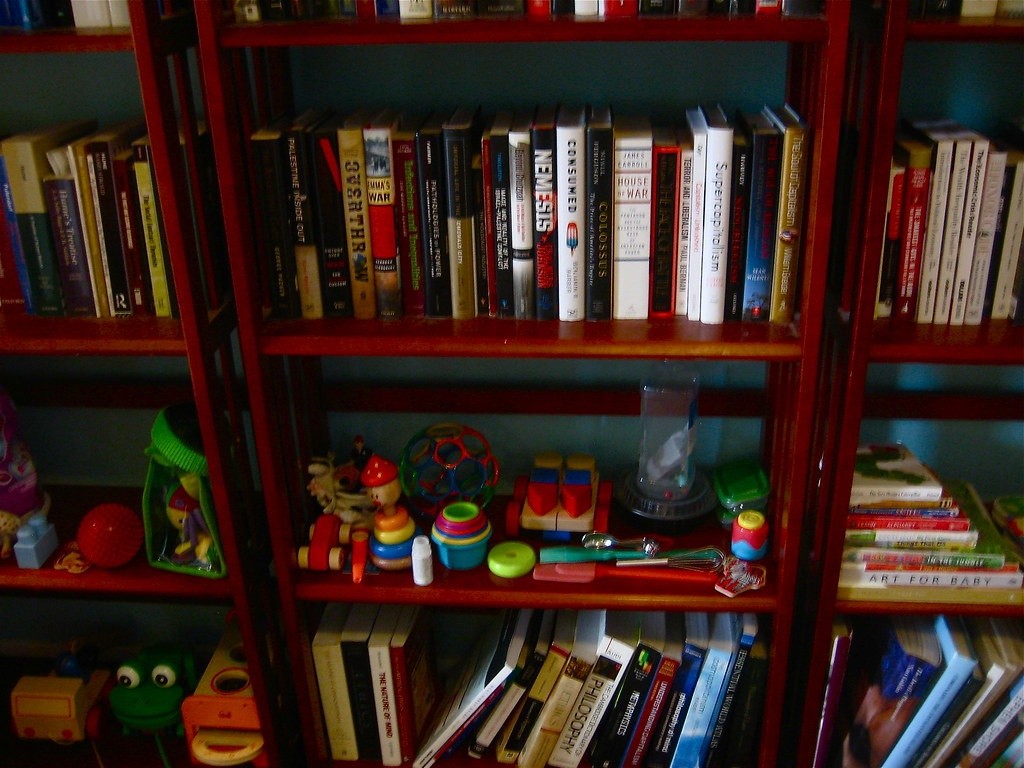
[812,611,1024,767]
[253,94,808,329]
[874,114,1022,325]
[832,442,1023,606]
[904,1,1022,22]
[1,1,132,30]
[309,606,767,767]
[0,117,175,318]
[224,0,821,22]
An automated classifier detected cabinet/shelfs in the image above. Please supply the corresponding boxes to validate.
[1,0,296,766]
[796,0,1023,768]
[183,0,843,768]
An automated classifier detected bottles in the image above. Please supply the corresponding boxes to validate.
[639,359,698,502]
